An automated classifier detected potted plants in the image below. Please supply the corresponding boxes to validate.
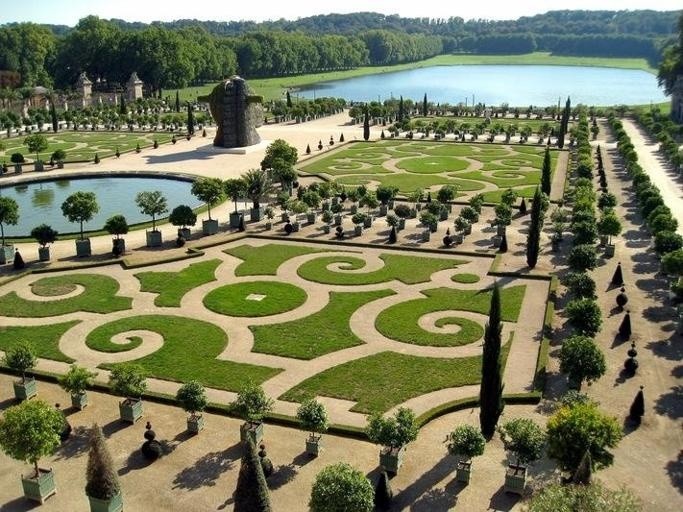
[495,417,546,497]
[0,340,39,401]
[107,363,148,425]
[2,129,67,175]
[84,421,125,512]
[233,434,274,512]
[295,400,330,457]
[363,406,421,475]
[167,203,198,241]
[442,424,486,485]
[556,191,626,394]
[32,224,59,261]
[135,189,169,247]
[227,378,277,446]
[60,191,99,257]
[1,398,69,504]
[190,139,301,235]
[56,363,99,412]
[263,181,519,246]
[308,463,376,512]
[1,195,20,263]
[106,215,131,254]
[176,380,208,436]
